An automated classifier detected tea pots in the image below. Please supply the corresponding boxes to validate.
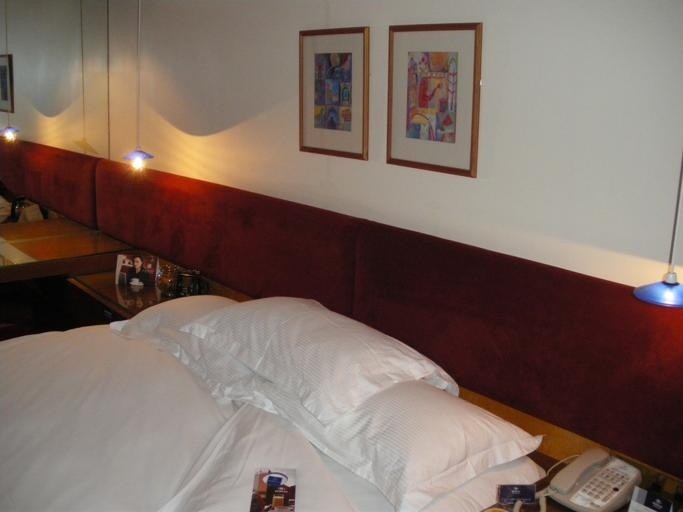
[11,194,26,222]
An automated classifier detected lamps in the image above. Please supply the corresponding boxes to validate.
[0,0,19,136]
[632,153,683,308]
[122,0,152,162]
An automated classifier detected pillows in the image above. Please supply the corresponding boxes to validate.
[249,355,542,509]
[121,295,261,403]
[178,296,460,433]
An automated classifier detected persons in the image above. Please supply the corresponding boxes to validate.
[126,256,147,287]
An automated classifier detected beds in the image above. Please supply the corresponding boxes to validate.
[2,295,545,512]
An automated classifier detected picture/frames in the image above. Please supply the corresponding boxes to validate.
[0,54,14,114]
[296,23,484,178]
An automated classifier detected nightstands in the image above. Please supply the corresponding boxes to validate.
[60,257,253,330]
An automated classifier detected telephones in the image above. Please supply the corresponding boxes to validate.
[548,447,643,512]
